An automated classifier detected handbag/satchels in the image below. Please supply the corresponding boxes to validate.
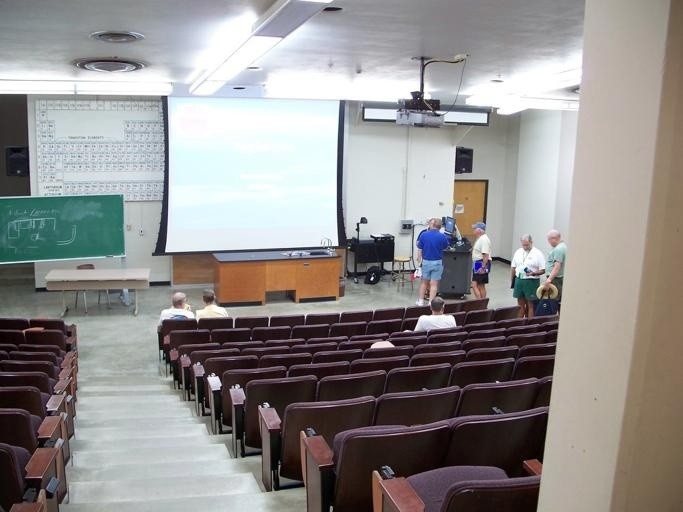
[532,288,558,317]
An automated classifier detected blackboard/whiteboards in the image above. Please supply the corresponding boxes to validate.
[0,193,126,264]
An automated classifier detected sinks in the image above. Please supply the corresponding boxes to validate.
[280,249,330,257]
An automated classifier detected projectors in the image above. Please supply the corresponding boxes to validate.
[396,108,444,127]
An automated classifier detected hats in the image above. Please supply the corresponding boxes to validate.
[470,222,486,231]
[534,283,558,301]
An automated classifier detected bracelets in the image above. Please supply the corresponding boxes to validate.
[547,278,551,282]
[480,266,485,270]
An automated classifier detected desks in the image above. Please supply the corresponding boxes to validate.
[211,249,342,307]
[437,237,472,296]
[45,269,150,318]
[345,241,394,279]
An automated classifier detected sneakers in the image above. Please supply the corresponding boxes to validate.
[415,298,432,307]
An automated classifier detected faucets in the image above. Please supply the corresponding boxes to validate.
[320,237,330,250]
[323,239,333,252]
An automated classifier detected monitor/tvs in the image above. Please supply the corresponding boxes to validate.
[445,216,456,234]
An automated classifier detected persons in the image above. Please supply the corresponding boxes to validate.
[413,296,458,332]
[470,222,491,299]
[510,232,545,315]
[154,292,195,336]
[195,289,229,323]
[542,229,566,302]
[415,218,449,307]
[416,218,436,301]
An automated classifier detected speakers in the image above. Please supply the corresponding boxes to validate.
[6,146,29,177]
[455,146,473,174]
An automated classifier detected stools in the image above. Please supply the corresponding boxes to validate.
[388,256,415,293]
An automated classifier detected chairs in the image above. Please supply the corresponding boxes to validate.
[0,316,83,512]
[446,407,549,459]
[73,265,107,314]
[156,298,561,458]
[299,404,453,512]
[371,458,544,512]
[258,395,377,492]
